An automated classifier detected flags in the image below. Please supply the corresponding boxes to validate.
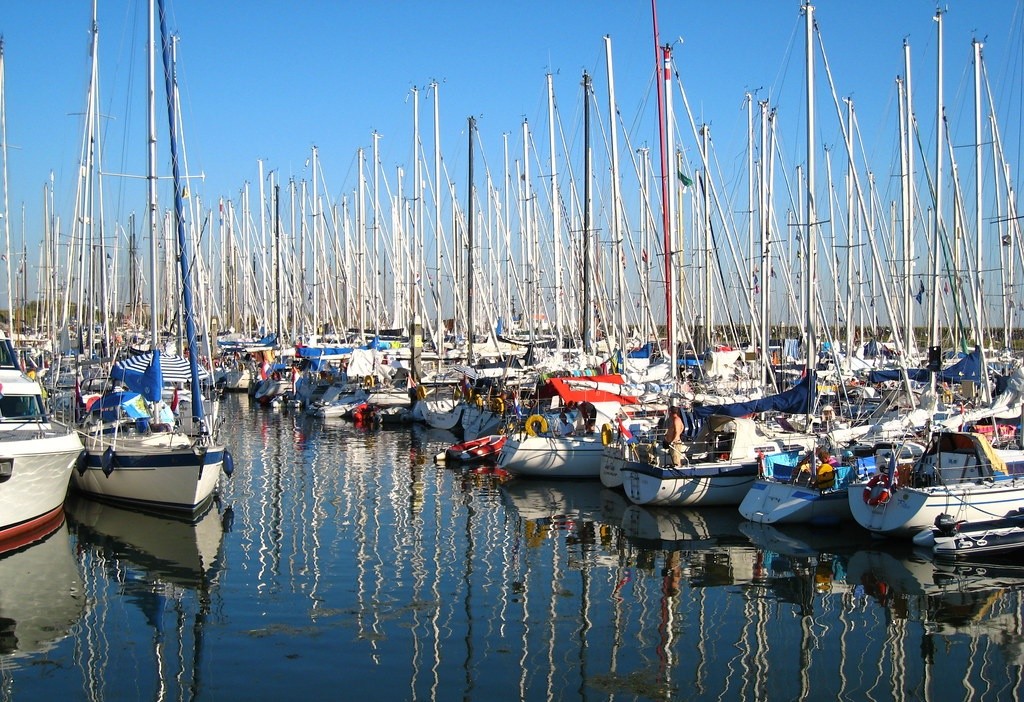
[618,419,639,446]
[679,167,693,187]
[75,372,85,411]
[916,280,926,305]
[172,392,181,416]
[292,367,300,384]
[462,374,471,390]
[260,361,273,380]
[513,395,523,419]
[409,378,417,399]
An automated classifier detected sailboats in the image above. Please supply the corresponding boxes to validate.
[0,0,1023,556]
[1,392,1024,701]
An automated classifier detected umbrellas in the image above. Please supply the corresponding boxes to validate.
[90,390,175,427]
[455,366,479,379]
[111,352,210,394]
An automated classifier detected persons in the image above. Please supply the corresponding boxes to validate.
[793,445,840,489]
[663,405,685,467]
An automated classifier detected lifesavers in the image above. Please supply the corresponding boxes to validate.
[863,475,891,505]
[364,375,374,389]
[528,520,547,545]
[491,397,505,413]
[943,392,953,405]
[525,414,547,437]
[816,464,835,489]
[879,581,891,607]
[475,394,483,408]
[601,425,613,446]
